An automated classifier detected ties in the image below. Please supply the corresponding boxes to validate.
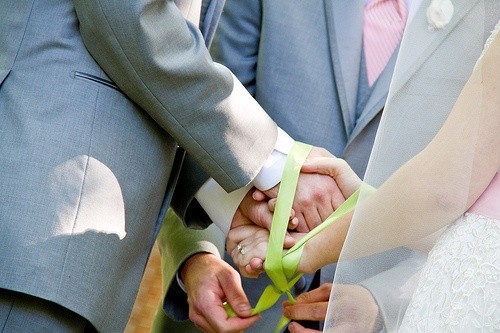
[363,0,407,87]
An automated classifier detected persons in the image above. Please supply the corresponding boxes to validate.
[0,0,347,333]
[151,0,500,333]
[227,21,499,333]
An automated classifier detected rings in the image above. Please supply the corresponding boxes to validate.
[240,248,246,255]
[236,243,242,252]
